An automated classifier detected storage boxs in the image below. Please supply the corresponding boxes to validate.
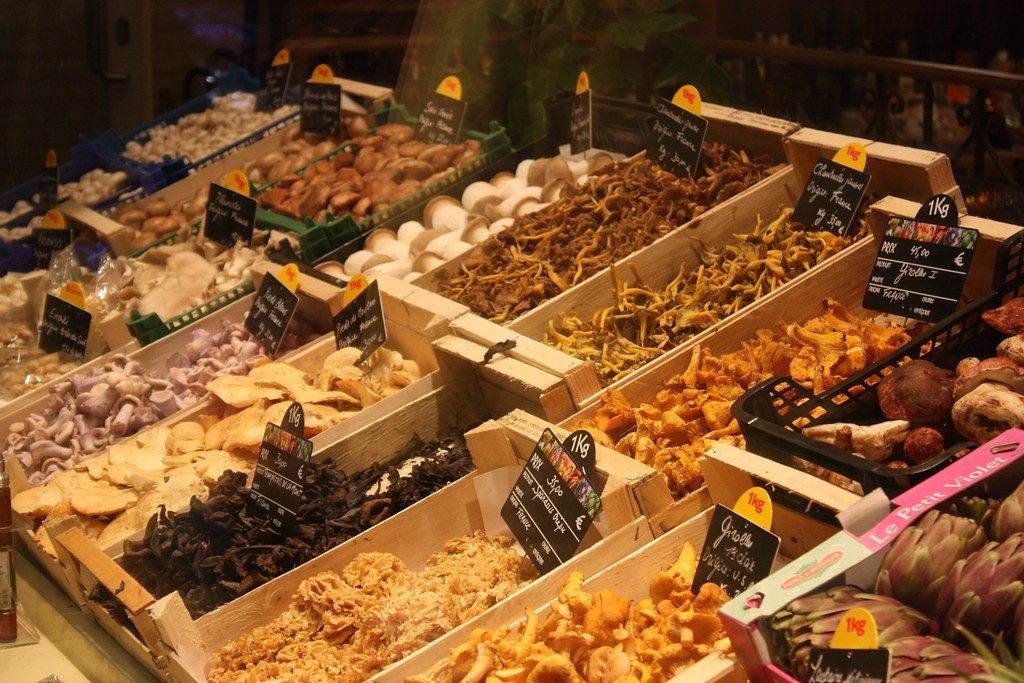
[0,67,1023,682]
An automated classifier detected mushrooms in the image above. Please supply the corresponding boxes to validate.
[0,90,1024,683]
